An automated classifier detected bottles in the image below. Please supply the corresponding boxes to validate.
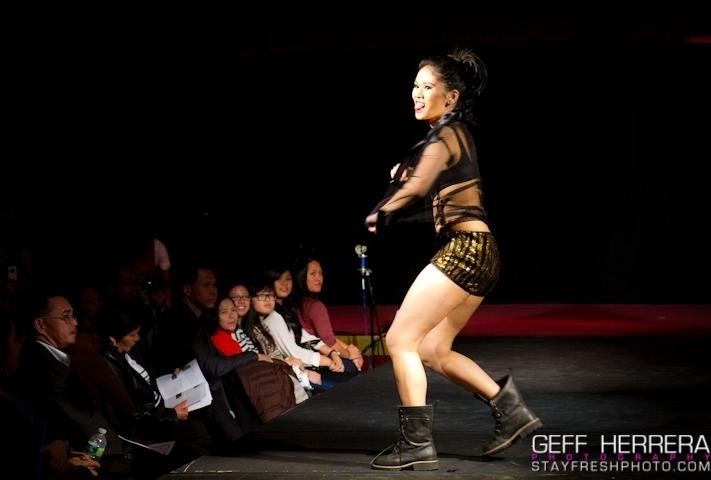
[81,427,108,470]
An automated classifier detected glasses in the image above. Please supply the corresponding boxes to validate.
[42,315,74,322]
[256,293,276,301]
[232,296,250,301]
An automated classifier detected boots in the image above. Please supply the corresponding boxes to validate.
[371,406,440,471]
[474,375,543,456]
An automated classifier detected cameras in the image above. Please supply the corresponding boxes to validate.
[144,276,155,290]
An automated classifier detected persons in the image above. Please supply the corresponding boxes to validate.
[360,46,543,471]
[2,229,367,480]
